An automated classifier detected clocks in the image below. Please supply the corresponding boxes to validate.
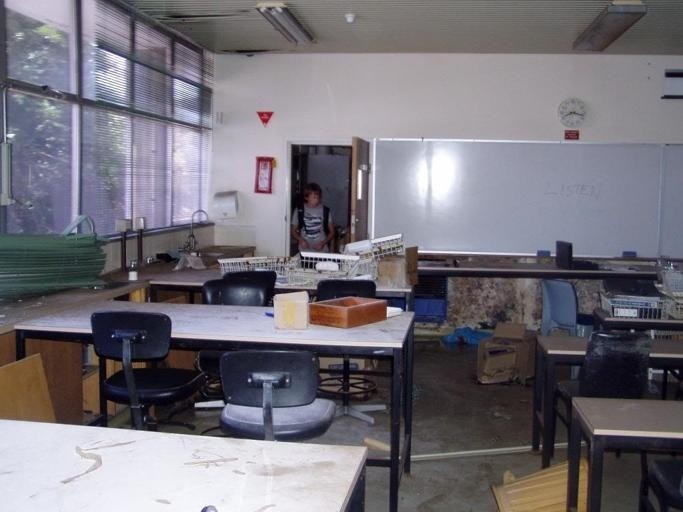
[556,97,587,129]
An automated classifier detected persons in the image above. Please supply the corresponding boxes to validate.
[290,181,335,252]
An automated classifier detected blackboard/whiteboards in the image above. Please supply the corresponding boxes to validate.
[371,136,683,260]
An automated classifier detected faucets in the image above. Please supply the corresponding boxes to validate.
[184,209,210,252]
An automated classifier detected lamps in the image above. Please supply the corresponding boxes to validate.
[570,1,646,54]
[254,2,314,48]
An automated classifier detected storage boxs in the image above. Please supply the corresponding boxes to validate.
[477,347,516,385]
[480,322,538,385]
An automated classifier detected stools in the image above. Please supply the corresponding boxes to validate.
[638,454,682,512]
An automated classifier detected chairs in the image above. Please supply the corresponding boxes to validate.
[312,277,390,424]
[89,307,205,429]
[191,279,271,392]
[550,331,652,458]
[541,279,593,336]
[218,348,336,444]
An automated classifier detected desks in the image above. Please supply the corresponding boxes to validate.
[1,418,368,512]
[148,260,416,309]
[12,297,417,512]
[532,332,682,465]
[593,307,682,332]
[567,396,682,512]
[417,255,661,308]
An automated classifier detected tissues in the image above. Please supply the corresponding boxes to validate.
[273,289,312,329]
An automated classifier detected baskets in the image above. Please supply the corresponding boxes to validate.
[599,290,683,320]
[217,233,404,289]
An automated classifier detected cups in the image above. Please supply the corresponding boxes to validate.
[128,271,137,280]
[285,270,348,288]
[377,275,410,290]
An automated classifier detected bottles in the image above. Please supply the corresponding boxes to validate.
[217,256,300,279]
[127,260,135,271]
[343,243,403,274]
[142,256,153,265]
[656,254,683,321]
[648,367,679,382]
[642,329,682,344]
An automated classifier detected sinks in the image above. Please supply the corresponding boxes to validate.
[196,250,225,268]
